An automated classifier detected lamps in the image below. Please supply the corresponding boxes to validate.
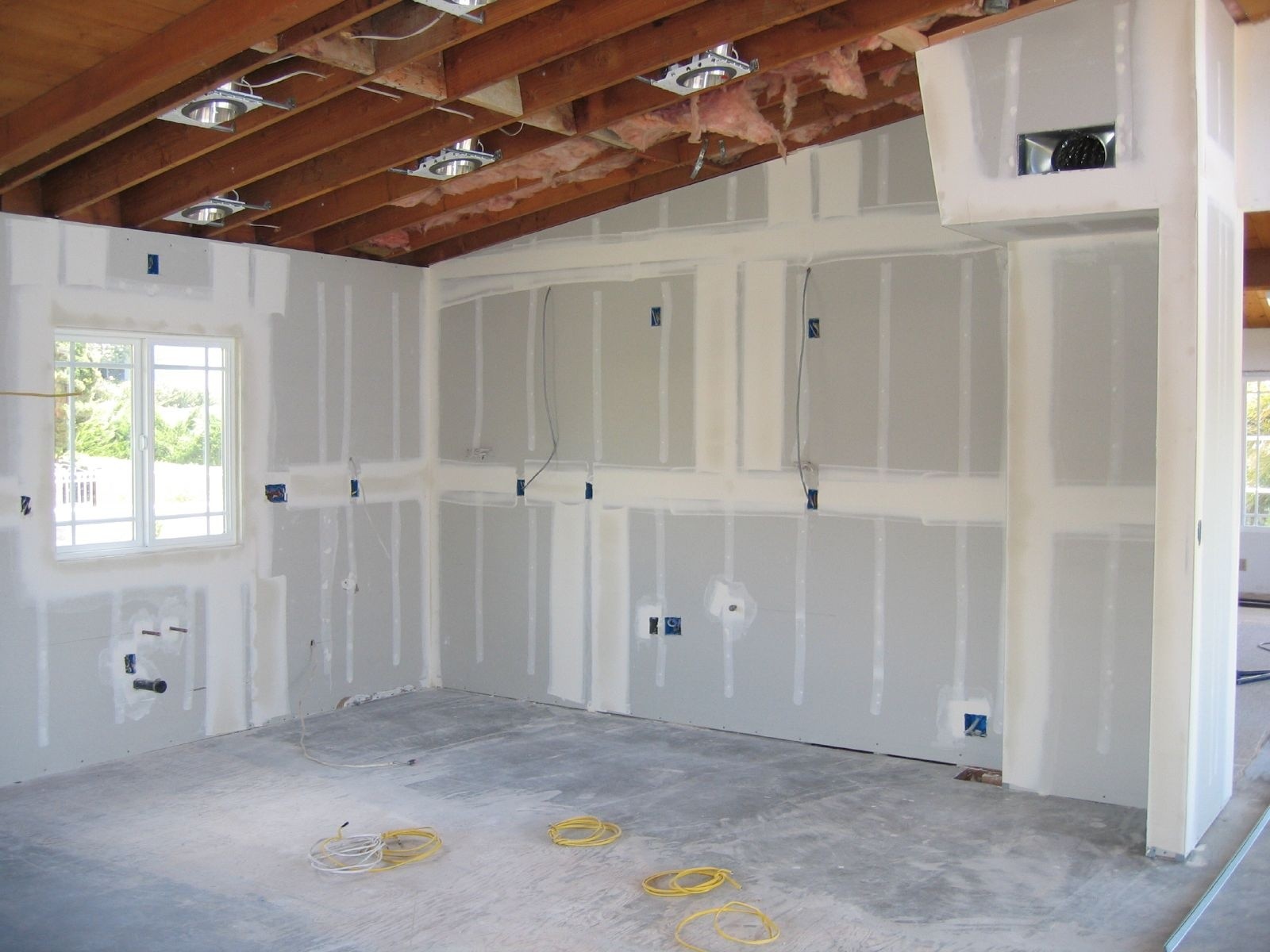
[648,42,759,95]
[414,1,498,25]
[391,133,502,182]
[155,77,298,133]
[163,191,267,230]
